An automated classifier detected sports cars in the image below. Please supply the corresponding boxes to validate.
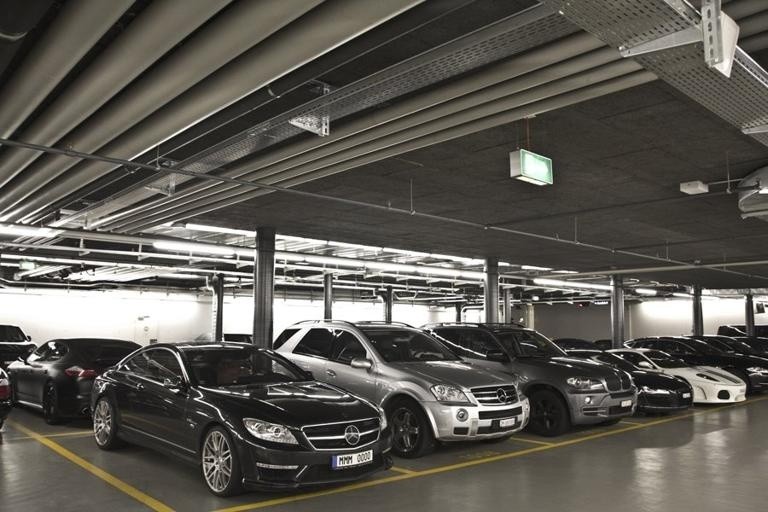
[562,349,694,418]
[604,349,746,405]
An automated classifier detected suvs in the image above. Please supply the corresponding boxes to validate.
[272,319,530,459]
[419,322,636,437]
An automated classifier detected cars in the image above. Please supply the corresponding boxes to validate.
[1,325,144,435]
[619,325,768,394]
[90,341,394,496]
[552,338,616,356]
